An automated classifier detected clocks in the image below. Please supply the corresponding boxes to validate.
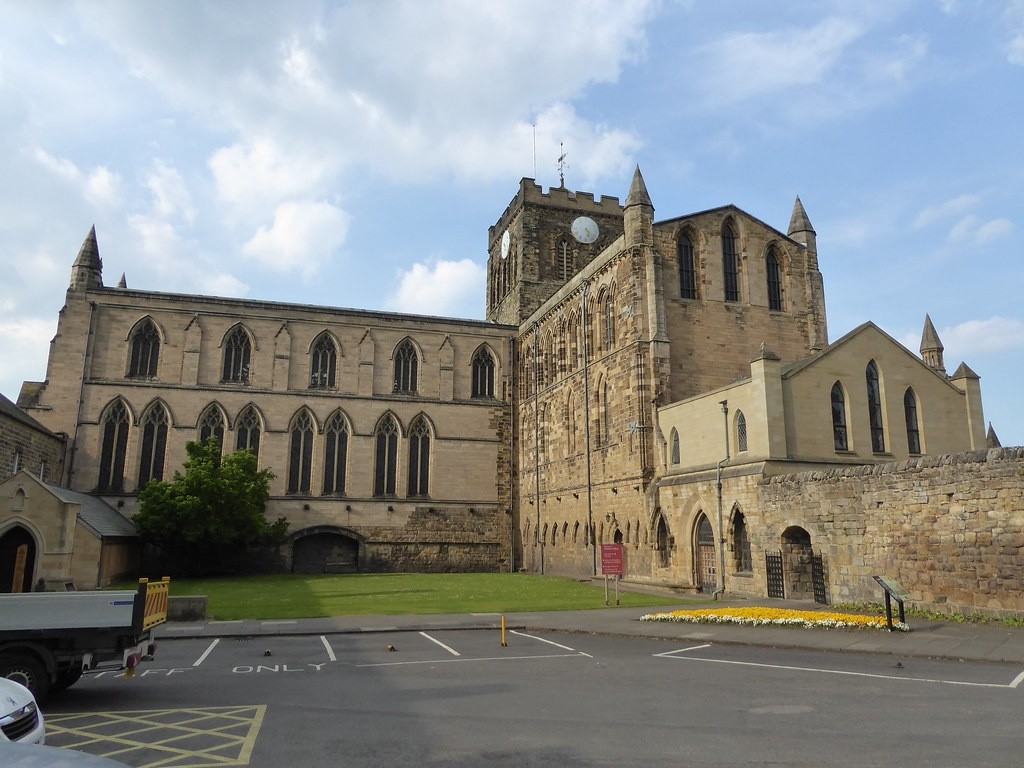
[501,231,510,259]
[571,216,599,244]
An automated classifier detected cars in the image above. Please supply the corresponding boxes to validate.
[1,674,45,750]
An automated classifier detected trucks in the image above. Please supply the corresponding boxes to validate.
[0,575,171,705]
